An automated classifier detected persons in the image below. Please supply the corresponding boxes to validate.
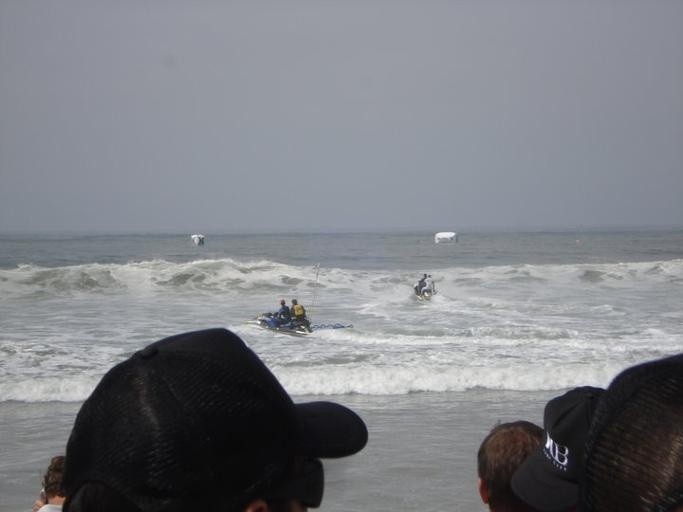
[421,275,434,296]
[34,456,66,512]
[419,272,426,292]
[276,298,289,320]
[289,297,308,321]
[475,420,547,511]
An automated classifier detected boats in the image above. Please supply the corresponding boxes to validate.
[434,231,457,246]
[191,234,204,246]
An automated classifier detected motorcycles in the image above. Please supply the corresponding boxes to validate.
[413,283,435,304]
[250,314,315,337]
